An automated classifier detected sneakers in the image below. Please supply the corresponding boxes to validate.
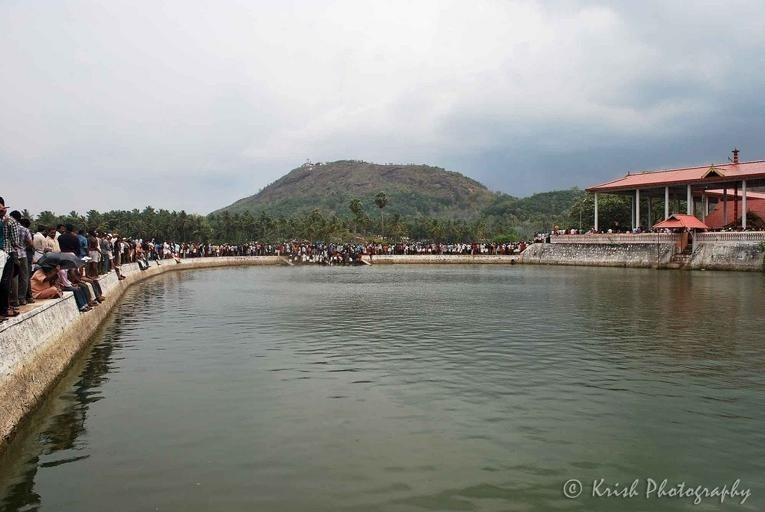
[141,265,151,270]
[118,275,126,280]
[80,294,106,313]
[0,296,35,324]
